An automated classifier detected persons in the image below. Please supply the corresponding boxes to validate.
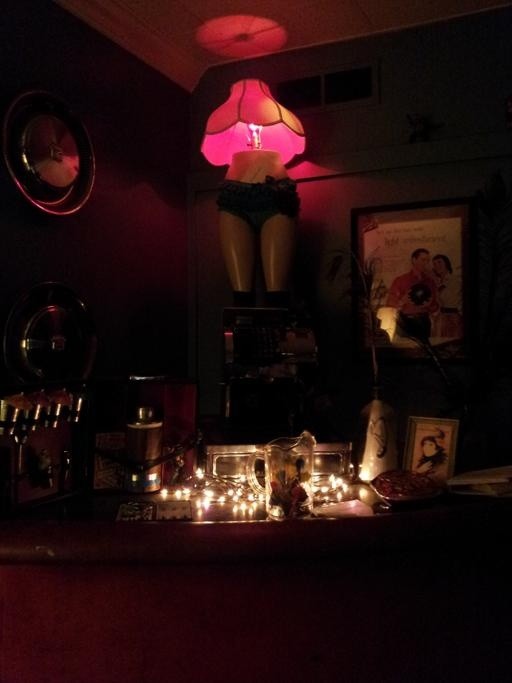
[430,254,461,338]
[415,430,446,477]
[215,150,302,295]
[386,247,440,337]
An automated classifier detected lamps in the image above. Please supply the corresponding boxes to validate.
[200,77,308,174]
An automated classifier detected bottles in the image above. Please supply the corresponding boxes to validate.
[0,390,91,440]
[125,405,164,494]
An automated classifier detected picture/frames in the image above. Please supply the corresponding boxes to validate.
[351,203,465,483]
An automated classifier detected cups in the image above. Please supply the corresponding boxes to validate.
[245,430,316,523]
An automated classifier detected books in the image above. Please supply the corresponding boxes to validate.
[447,465,512,487]
[446,483,512,497]
